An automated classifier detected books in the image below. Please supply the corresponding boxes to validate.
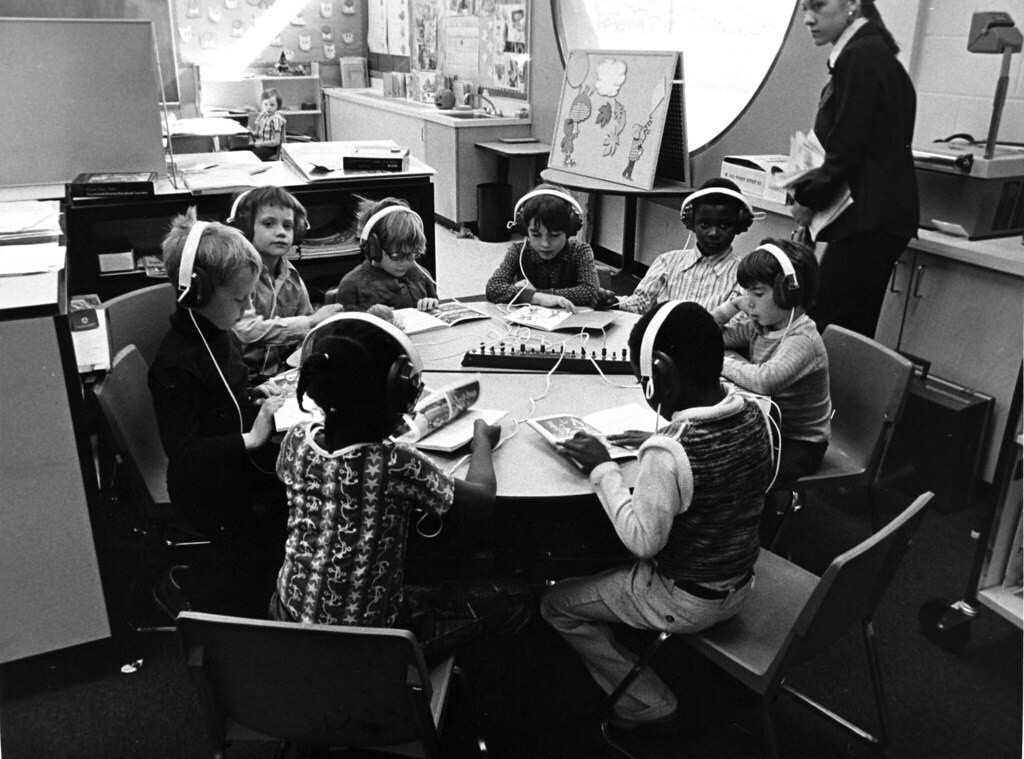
[392,303,490,334]
[505,304,623,331]
[271,376,326,432]
[526,403,670,469]
[288,232,361,260]
[388,379,510,451]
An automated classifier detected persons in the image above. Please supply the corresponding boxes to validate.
[541,301,773,759]
[709,238,832,561]
[228,185,344,380]
[147,207,284,612]
[247,89,283,160]
[271,321,535,696]
[791,0,920,339]
[336,197,439,320]
[486,184,599,315]
[589,178,754,314]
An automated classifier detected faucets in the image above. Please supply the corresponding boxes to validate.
[463,92,502,117]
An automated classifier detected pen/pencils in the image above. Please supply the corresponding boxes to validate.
[204,163,219,170]
[250,169,267,177]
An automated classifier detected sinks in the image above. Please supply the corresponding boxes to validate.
[438,109,514,120]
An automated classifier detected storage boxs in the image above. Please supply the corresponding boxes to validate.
[721,155,792,204]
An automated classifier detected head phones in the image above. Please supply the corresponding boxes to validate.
[638,300,696,408]
[172,220,211,310]
[507,189,584,237]
[227,189,311,242]
[679,187,767,235]
[359,205,424,263]
[755,243,804,310]
[284,311,425,420]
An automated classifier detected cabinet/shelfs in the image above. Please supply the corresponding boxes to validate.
[325,97,531,231]
[729,206,1024,631]
[65,172,435,308]
[256,62,326,143]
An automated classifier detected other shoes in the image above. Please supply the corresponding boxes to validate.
[152,564,199,626]
[608,708,679,736]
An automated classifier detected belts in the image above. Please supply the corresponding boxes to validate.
[663,567,754,600]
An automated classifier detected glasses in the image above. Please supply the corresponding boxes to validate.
[381,247,421,261]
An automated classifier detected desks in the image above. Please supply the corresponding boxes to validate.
[474,142,551,184]
[275,302,770,658]
[539,167,696,288]
[170,117,249,152]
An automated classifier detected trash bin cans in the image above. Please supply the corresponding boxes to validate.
[475,183,510,242]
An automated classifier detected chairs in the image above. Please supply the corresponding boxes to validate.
[95,263,931,758]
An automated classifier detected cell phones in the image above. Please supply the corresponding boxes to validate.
[71,308,99,332]
